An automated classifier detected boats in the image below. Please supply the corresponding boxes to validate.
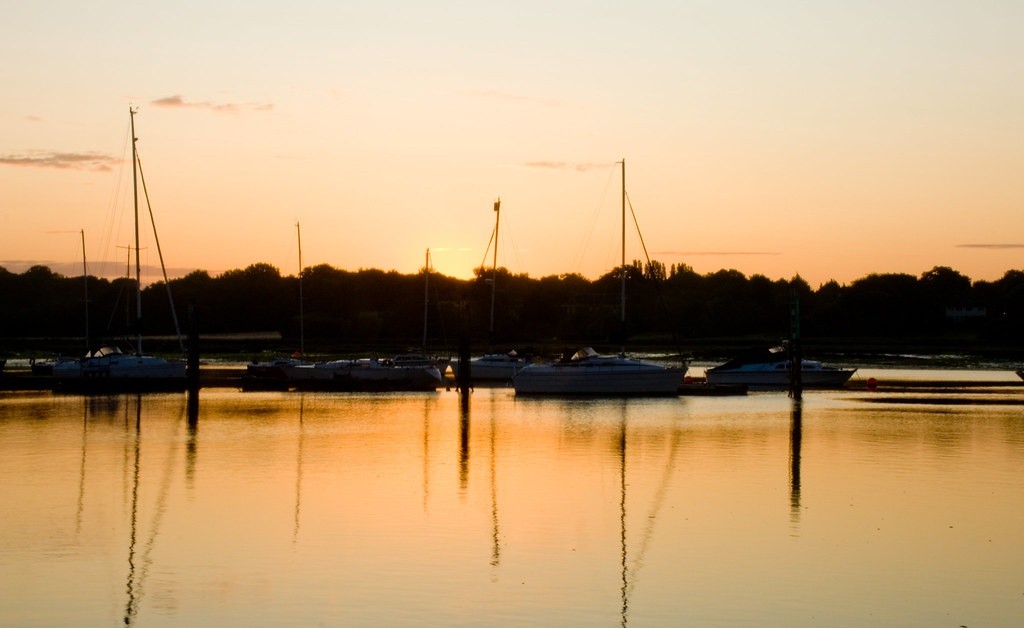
[703,338,860,388]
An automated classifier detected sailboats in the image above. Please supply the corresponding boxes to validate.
[28,106,187,391]
[276,202,525,390]
[511,159,689,399]
